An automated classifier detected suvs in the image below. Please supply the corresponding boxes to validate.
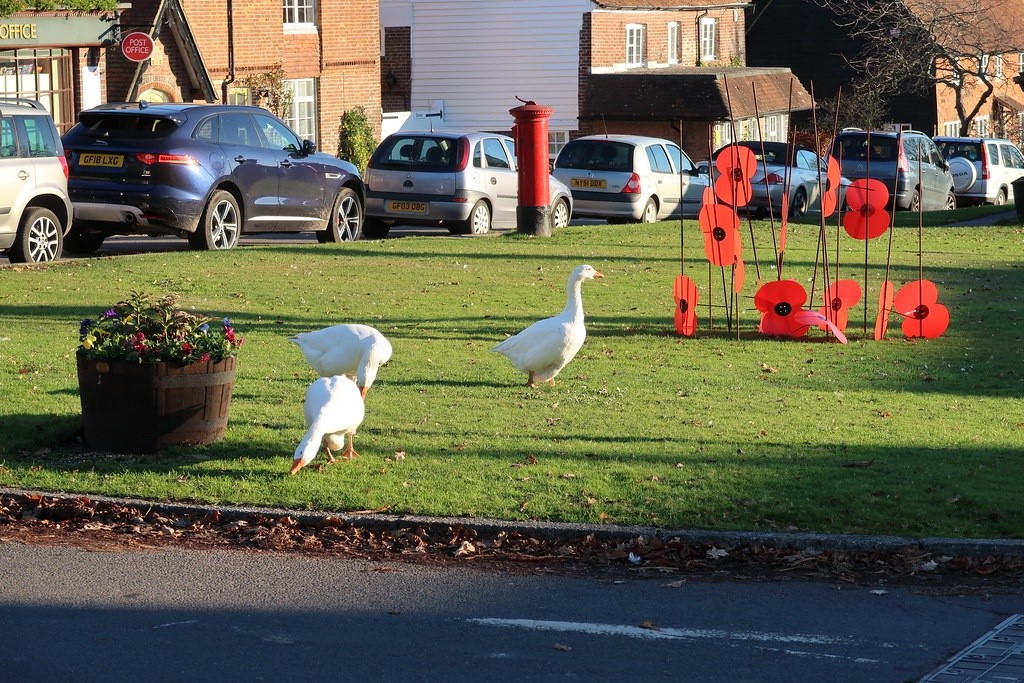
[59,99,368,256]
[930,132,1024,209]
[0,95,72,268]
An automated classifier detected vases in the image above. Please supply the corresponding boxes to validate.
[75,353,237,447]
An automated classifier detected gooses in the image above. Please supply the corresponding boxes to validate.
[490,265,606,389]
[287,375,366,475]
[287,324,393,402]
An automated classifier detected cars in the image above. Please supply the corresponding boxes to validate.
[355,125,574,247]
[696,137,855,223]
[551,132,717,228]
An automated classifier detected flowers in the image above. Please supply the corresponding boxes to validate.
[74,290,242,365]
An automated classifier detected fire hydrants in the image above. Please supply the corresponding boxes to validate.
[506,96,554,240]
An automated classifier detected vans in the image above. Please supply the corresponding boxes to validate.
[822,126,957,216]
